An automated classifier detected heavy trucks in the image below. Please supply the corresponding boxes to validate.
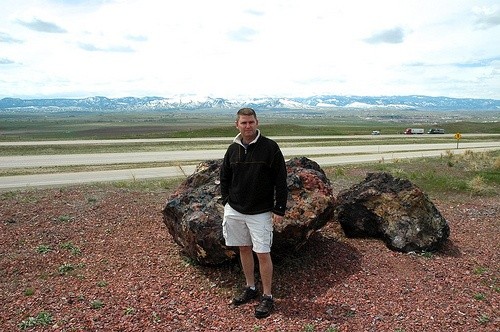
[428,128,444,134]
[404,128,424,134]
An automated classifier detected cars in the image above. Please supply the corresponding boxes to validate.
[372,130,379,135]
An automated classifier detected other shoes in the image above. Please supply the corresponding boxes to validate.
[232,285,260,306]
[254,294,274,317]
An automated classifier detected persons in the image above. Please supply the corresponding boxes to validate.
[220,108,289,318]
